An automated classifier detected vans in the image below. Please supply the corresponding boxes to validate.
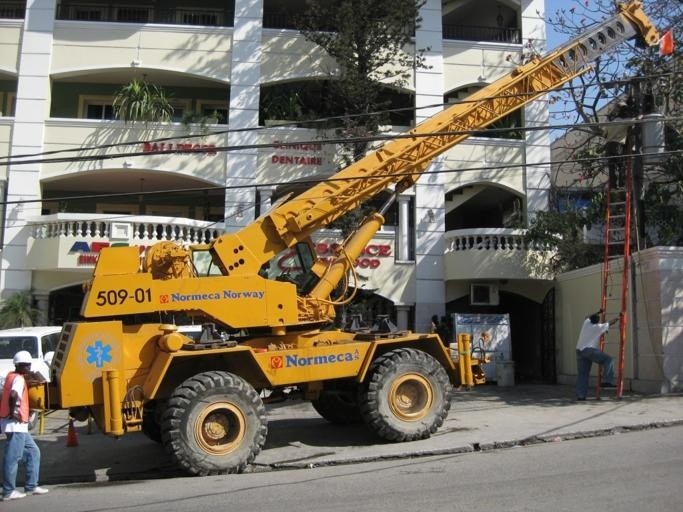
[1,327,64,429]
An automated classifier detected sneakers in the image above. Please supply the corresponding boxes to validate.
[26,487,49,496]
[598,382,618,389]
[3,491,27,501]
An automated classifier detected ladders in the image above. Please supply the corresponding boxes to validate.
[595,157,637,400]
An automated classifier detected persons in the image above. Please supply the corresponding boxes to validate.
[603,108,642,191]
[0,349,48,500]
[571,306,623,402]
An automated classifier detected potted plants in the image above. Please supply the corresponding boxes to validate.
[185,110,224,139]
[264,93,299,128]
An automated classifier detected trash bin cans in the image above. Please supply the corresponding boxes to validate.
[496,360,516,387]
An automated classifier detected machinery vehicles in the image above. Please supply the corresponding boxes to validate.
[28,0,682,476]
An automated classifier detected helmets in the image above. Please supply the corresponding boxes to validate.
[13,351,36,365]
